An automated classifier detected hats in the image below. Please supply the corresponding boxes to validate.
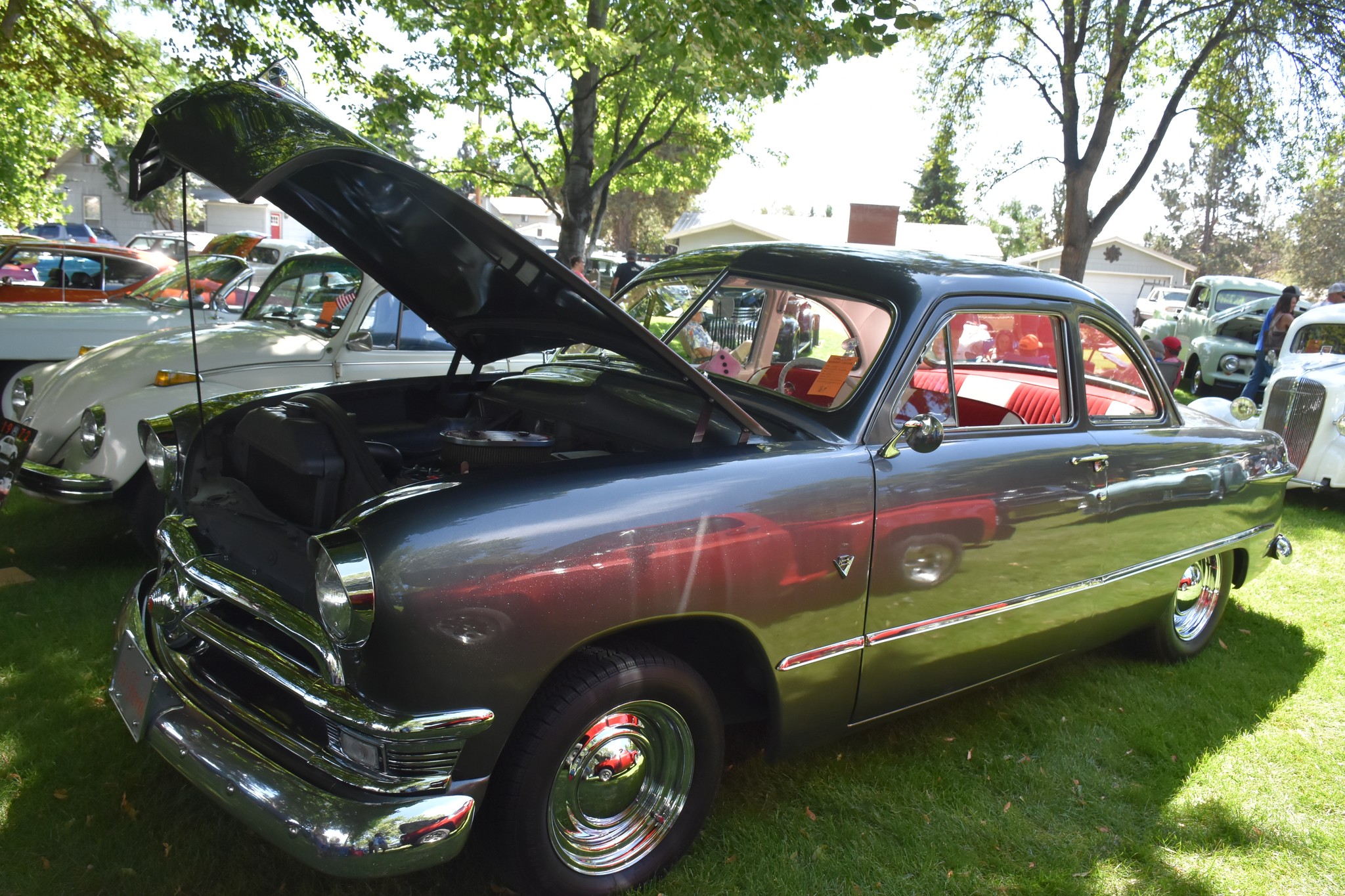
[1019,334,1043,350]
[1283,286,1305,296]
[627,248,637,256]
[1144,338,1166,355]
[1328,282,1345,293]
[683,300,707,313]
[1163,337,1182,352]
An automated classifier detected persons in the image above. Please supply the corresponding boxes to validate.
[1237,285,1305,402]
[1266,294,1298,358]
[609,247,646,300]
[991,332,1021,362]
[307,276,331,304]
[682,299,735,358]
[1161,336,1185,395]
[997,334,1054,370]
[1305,282,1345,312]
[568,256,598,290]
[964,338,996,362]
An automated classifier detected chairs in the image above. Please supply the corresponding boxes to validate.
[678,330,716,365]
[1138,359,1186,393]
[68,271,94,289]
[168,243,176,255]
[43,269,70,288]
[1003,351,1051,369]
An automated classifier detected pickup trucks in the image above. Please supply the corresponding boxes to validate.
[1141,275,1311,396]
[400,494,998,678]
[1134,287,1201,327]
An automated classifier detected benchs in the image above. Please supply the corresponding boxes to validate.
[745,363,1028,428]
[908,369,1157,426]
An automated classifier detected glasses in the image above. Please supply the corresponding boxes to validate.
[1341,294,1345,299]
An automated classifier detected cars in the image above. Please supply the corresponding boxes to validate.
[1186,304,1345,494]
[103,77,1300,896]
[988,448,1251,525]
[2,220,820,507]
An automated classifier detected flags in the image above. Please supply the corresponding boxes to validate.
[334,288,357,311]
